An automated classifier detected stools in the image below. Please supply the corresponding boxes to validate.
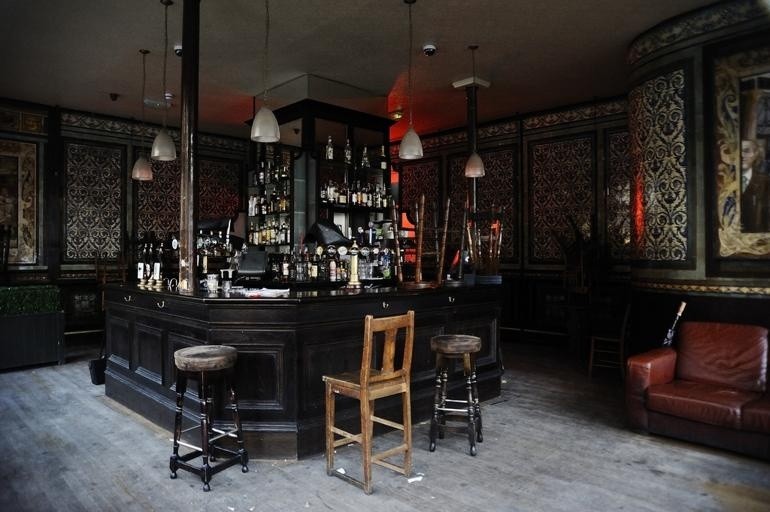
[428,332,485,457]
[386,191,507,290]
[167,340,250,489]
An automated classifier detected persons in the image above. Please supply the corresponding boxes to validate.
[741,139,769,233]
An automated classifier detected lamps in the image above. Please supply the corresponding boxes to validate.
[250,1,280,145]
[463,41,485,178]
[149,0,179,165]
[398,1,424,161]
[132,46,154,183]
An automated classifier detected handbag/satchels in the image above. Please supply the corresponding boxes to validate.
[89,358,106,385]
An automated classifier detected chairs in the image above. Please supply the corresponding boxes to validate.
[538,215,639,381]
[61,229,184,360]
[319,309,416,494]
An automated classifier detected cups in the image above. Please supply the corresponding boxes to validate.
[223,280,233,294]
[207,274,219,293]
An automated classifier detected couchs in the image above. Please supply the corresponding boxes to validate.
[625,318,768,455]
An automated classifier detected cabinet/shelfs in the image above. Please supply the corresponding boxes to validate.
[244,140,308,249]
[309,108,390,249]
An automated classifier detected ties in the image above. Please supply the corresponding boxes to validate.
[741,176,748,193]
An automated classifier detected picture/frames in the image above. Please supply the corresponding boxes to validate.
[741,69,770,234]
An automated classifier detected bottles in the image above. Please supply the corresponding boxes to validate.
[324,134,387,169]
[267,243,398,286]
[338,219,395,244]
[246,162,291,245]
[320,180,393,209]
[138,242,166,282]
